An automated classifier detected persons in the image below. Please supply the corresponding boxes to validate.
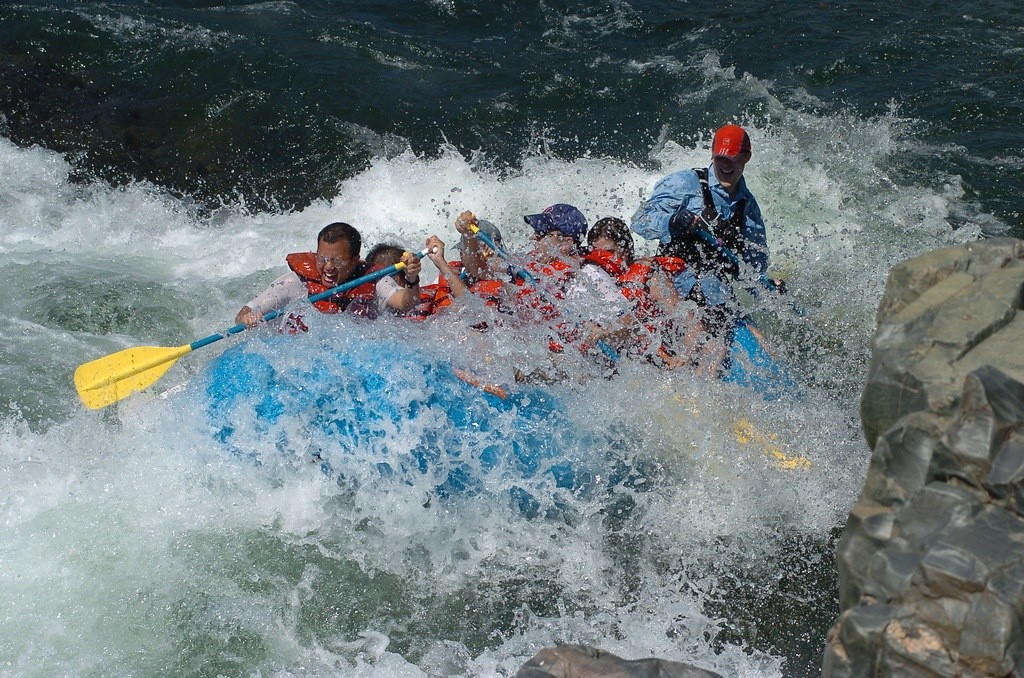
[236,204,778,399]
[630,124,770,317]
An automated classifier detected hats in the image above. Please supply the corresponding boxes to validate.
[524,204,588,245]
[711,125,751,160]
[449,220,501,249]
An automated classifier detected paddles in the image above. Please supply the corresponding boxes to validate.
[73,245,437,413]
[466,220,808,470]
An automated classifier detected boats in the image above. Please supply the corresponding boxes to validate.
[199,312,816,508]
